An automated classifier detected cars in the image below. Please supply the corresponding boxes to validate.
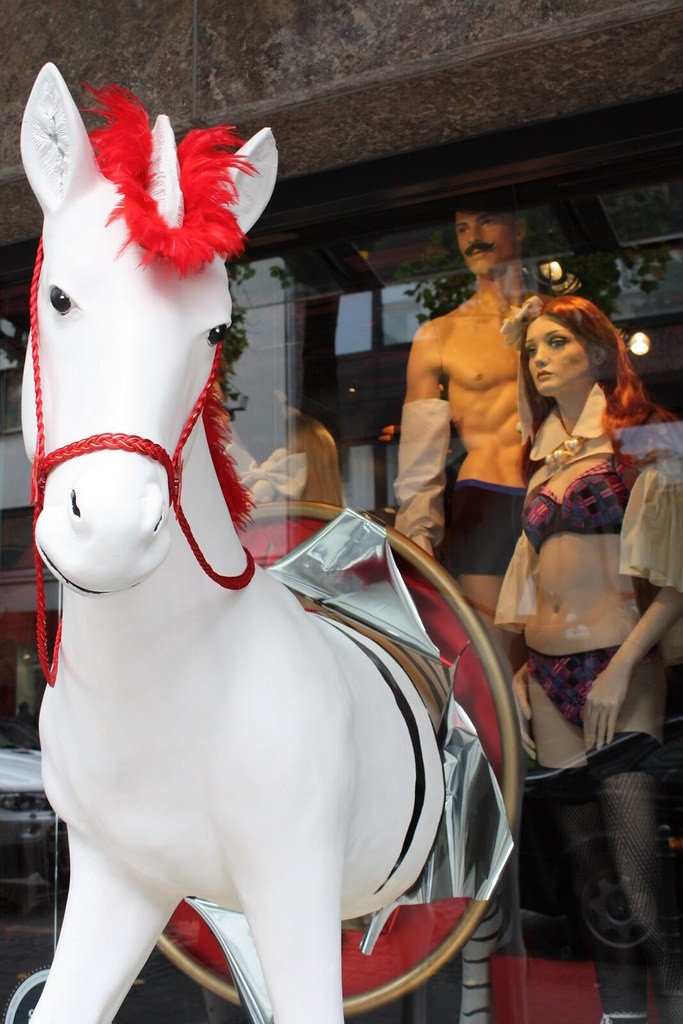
[0,729,70,890]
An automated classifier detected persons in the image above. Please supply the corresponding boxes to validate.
[393,188,556,690]
[493,293,683,1024]
[7,702,38,749]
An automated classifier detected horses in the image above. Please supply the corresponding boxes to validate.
[19,62,444,1024]
